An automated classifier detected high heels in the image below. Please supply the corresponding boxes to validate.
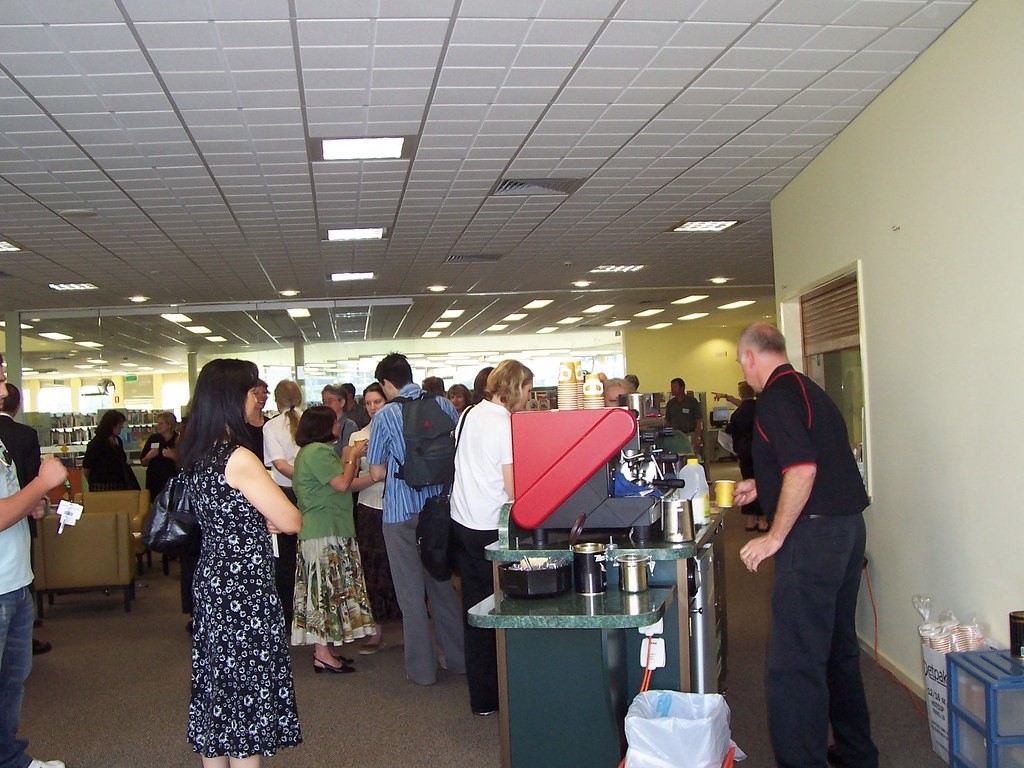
[312,655,355,673]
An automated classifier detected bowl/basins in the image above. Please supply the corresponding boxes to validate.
[499,561,571,595]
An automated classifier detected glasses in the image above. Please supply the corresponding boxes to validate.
[0,445,13,467]
[322,398,340,404]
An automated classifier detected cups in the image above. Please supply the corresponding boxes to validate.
[716,480,736,508]
[573,542,606,596]
[1010,611,1024,657]
[920,619,982,655]
[557,361,605,411]
[664,500,696,542]
[615,555,652,592]
[355,438,366,446]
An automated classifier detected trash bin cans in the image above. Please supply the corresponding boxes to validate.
[623,688,733,768]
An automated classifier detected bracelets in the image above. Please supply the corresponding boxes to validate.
[724,394,727,398]
[345,461,357,468]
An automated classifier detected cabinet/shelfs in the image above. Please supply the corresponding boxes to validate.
[945,649,1024,768]
[24,408,174,462]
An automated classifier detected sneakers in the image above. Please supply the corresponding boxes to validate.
[29,758,65,768]
[359,639,387,654]
[827,744,879,768]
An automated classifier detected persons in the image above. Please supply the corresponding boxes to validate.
[82,409,141,492]
[454,359,535,717]
[712,381,771,534]
[175,354,640,675]
[367,352,465,686]
[664,377,712,486]
[140,412,181,501]
[178,358,303,768]
[731,321,881,768]
[0,354,68,768]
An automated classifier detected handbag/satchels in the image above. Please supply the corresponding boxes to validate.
[416,493,451,583]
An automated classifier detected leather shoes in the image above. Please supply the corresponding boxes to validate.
[32,638,52,654]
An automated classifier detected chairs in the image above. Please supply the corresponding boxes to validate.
[74,489,151,573]
[33,512,136,618]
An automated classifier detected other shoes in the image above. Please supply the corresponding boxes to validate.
[744,521,770,532]
[437,654,447,668]
[184,620,193,632]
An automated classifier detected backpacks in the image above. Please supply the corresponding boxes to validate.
[385,390,458,493]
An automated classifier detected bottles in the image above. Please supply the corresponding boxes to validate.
[673,459,710,525]
